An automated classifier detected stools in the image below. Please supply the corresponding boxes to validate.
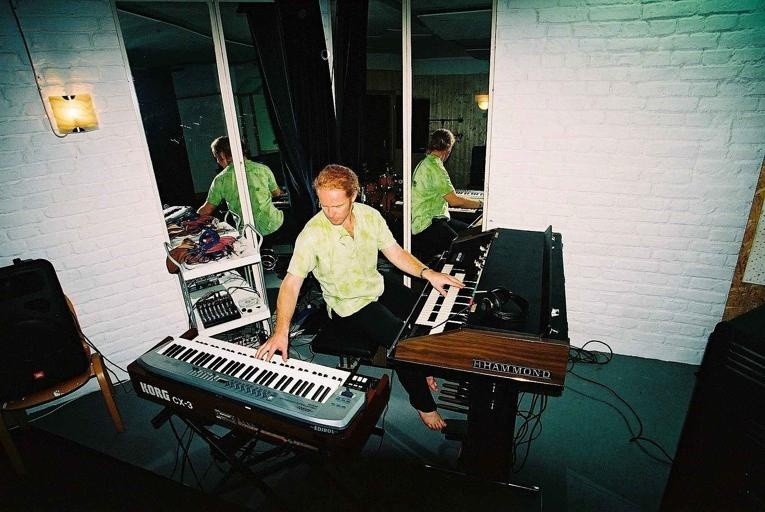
[308,332,379,374]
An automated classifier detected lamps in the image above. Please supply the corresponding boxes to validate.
[49,93,100,135]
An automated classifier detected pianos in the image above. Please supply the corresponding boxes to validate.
[136,334,379,429]
[448,190,483,214]
[386,224,570,389]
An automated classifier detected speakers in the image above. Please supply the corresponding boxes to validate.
[658,304,765,512]
[0,258,88,402]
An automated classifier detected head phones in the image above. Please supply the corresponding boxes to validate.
[480,287,532,322]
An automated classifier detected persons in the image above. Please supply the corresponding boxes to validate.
[411,128,484,253]
[254,164,467,432]
[195,135,309,252]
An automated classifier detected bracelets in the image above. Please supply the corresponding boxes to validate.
[420,268,429,280]
[479,200,482,208]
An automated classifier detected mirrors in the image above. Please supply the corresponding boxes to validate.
[112,1,488,315]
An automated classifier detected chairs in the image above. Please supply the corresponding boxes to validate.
[0,293,125,479]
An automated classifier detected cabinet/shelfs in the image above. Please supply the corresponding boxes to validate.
[166,224,272,351]
[148,402,381,511]
